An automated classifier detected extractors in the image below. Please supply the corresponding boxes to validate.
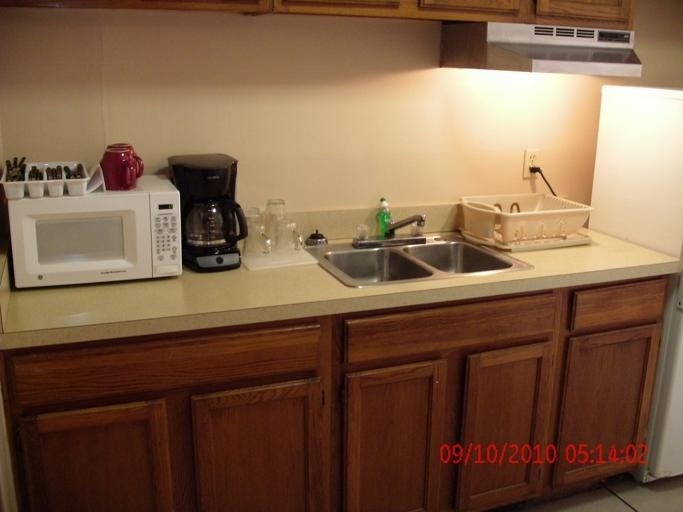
[439,20,644,81]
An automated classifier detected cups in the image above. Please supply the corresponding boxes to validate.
[246,191,304,258]
[99,140,146,190]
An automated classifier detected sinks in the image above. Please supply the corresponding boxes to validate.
[304,243,433,289]
[394,232,536,279]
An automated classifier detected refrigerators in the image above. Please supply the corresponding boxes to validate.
[584,85,683,486]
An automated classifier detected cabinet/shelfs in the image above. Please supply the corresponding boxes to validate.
[275,0,525,29]
[81,1,275,14]
[551,279,669,501]
[336,291,563,510]
[0,315,337,512]
[525,0,635,28]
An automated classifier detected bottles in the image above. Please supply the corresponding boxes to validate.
[374,196,394,240]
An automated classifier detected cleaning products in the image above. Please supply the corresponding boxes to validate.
[375,198,392,236]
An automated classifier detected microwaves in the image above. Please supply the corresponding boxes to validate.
[3,173,181,290]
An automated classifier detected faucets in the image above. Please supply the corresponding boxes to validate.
[386,213,426,236]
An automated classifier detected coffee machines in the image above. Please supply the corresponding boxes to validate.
[166,152,248,273]
